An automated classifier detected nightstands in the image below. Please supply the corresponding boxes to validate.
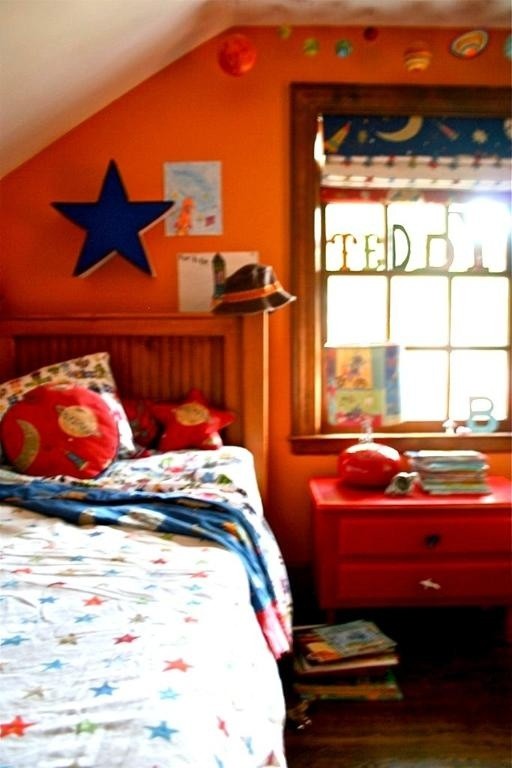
[305,472,511,624]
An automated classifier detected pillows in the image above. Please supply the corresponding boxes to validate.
[0,351,238,479]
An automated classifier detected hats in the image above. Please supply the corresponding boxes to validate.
[212,263,298,316]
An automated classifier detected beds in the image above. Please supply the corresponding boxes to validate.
[0,315,293,768]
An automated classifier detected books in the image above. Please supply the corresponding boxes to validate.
[294,619,404,702]
[403,449,491,495]
[323,345,401,426]
